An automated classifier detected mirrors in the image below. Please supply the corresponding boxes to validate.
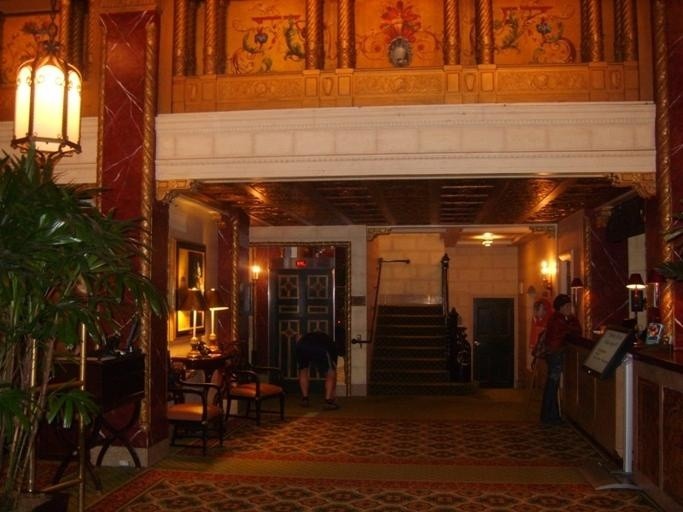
[173,236,207,340]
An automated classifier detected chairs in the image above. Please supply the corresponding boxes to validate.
[220,340,287,431]
[165,355,226,460]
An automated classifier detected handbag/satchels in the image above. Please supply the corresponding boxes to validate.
[531,329,545,359]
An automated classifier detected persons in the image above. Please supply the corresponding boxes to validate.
[537,293,580,424]
[292,330,340,412]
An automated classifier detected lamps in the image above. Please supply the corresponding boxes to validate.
[624,270,647,348]
[10,1,89,162]
[568,277,584,303]
[248,266,260,286]
[175,284,209,360]
[203,286,230,355]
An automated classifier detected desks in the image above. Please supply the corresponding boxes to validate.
[48,348,147,491]
[173,350,233,437]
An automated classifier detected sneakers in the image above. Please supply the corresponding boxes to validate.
[323,398,338,410]
[300,396,310,408]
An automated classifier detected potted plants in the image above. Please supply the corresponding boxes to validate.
[0,144,169,512]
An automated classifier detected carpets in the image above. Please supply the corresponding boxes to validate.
[173,408,619,473]
[79,461,667,511]
